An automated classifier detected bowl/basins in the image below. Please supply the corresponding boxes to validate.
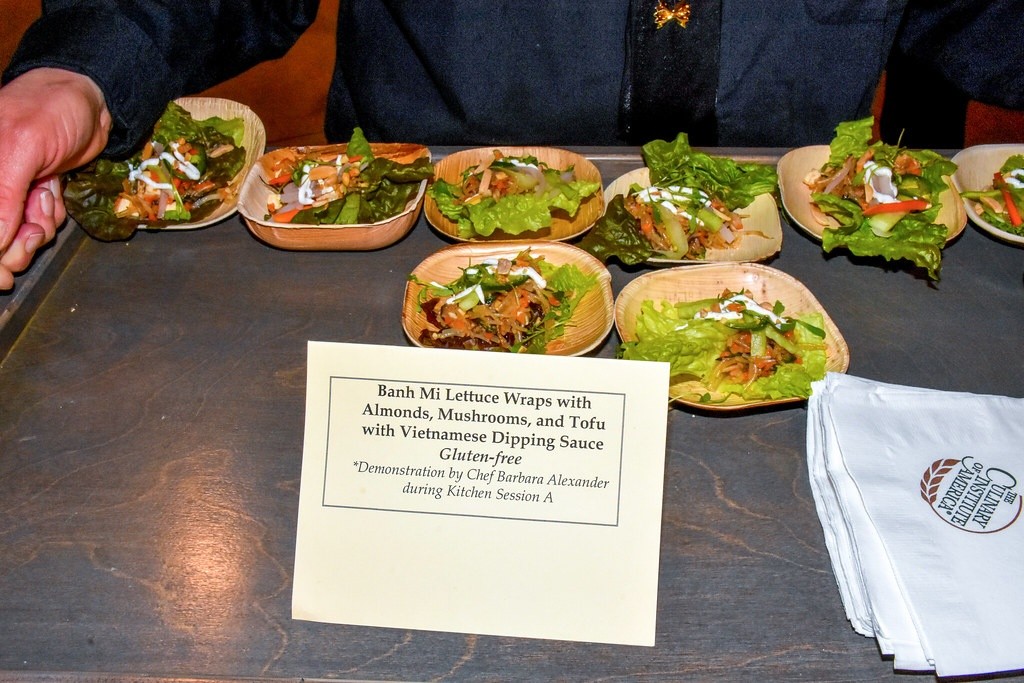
[236,143,431,251]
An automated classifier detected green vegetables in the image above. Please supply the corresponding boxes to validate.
[62,101,246,241]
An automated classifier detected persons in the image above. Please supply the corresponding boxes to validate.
[0,0,1024,290]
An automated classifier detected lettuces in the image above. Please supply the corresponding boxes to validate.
[266,116,1024,405]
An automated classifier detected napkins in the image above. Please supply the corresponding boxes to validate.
[804,369,1024,683]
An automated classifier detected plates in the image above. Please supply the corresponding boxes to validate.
[424,147,605,244]
[135,97,266,229]
[615,263,850,410]
[402,241,615,356]
[777,145,968,241]
[949,144,1024,244]
[603,166,783,264]
